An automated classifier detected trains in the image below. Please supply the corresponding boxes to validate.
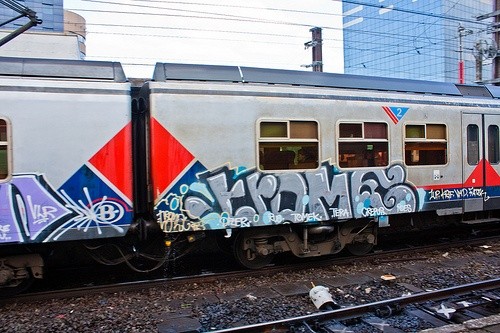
[0,0,500,293]
